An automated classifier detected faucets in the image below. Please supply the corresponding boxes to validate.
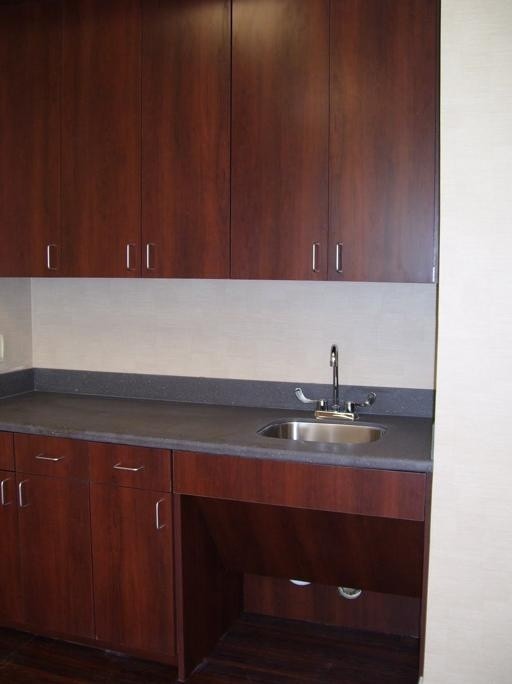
[329,344,341,409]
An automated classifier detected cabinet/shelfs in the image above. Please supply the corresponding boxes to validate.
[0,428,96,649]
[0,0,61,283]
[60,1,231,280]
[231,1,441,284]
[87,439,178,667]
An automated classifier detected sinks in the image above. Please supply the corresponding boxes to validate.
[257,417,390,447]
